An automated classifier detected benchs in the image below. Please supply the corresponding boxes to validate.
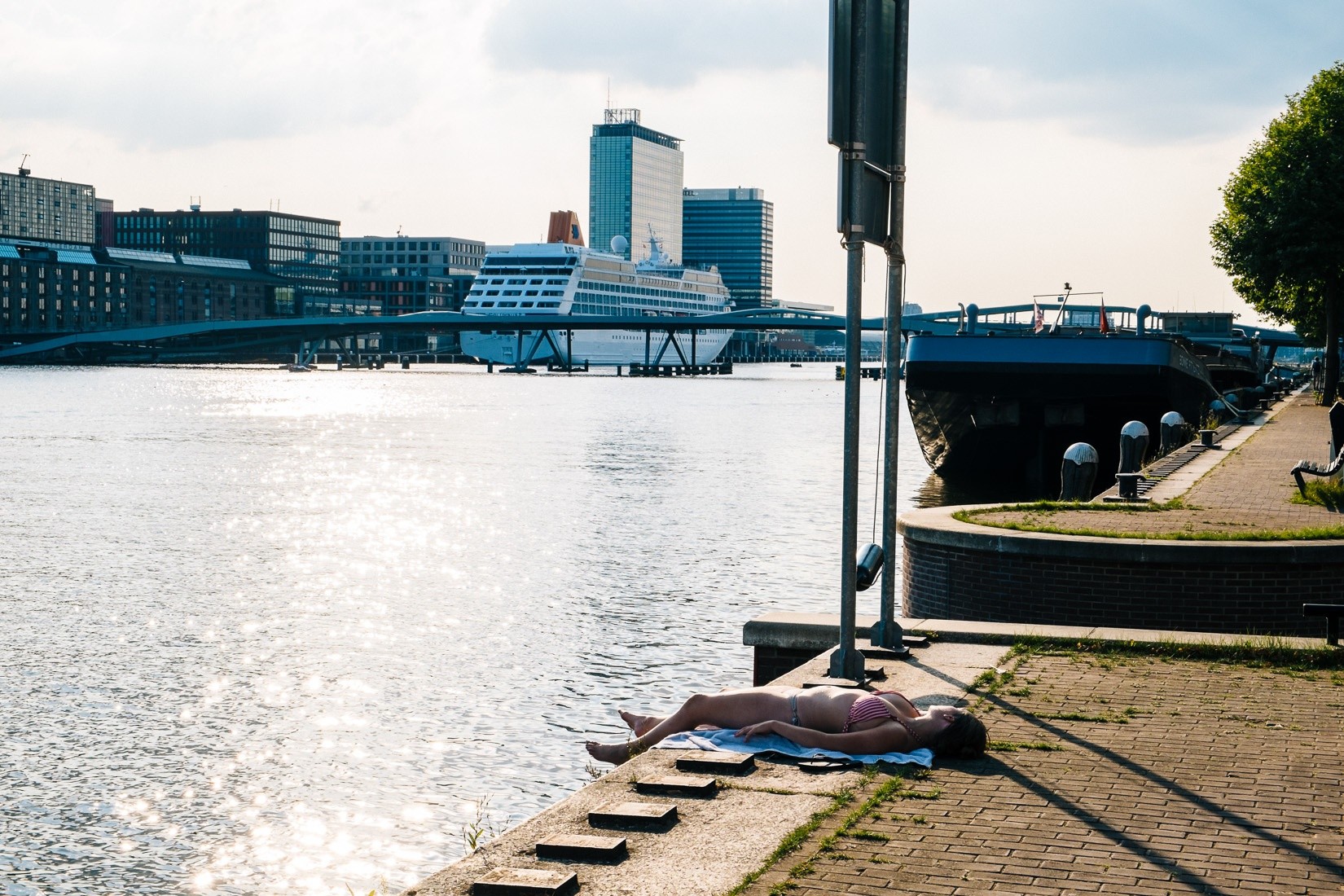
[1290,443,1344,500]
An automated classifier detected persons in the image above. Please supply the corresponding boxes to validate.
[583,684,990,768]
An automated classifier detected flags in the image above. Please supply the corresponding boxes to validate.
[1100,298,1109,334]
[1034,300,1044,333]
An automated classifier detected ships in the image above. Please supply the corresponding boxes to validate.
[459,223,735,366]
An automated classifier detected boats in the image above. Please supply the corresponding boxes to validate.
[790,363,802,367]
[644,310,657,317]
[904,283,1305,501]
[660,312,672,317]
[676,313,688,317]
